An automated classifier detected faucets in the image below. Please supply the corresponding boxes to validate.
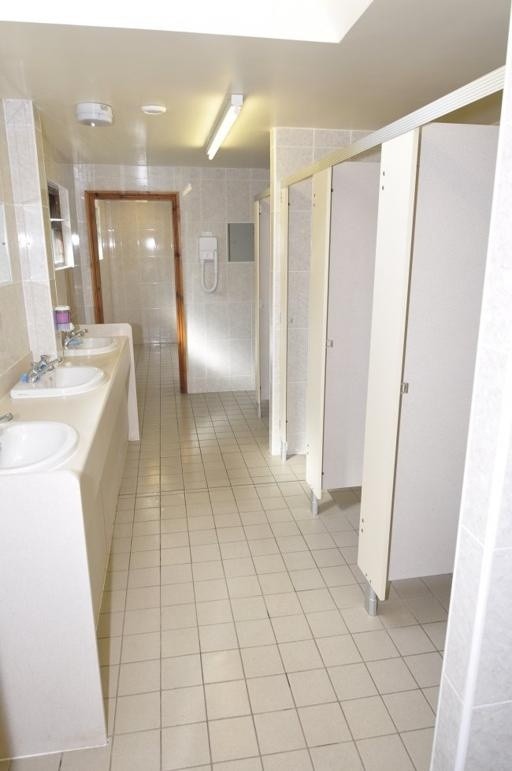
[33,354,62,365]
[31,365,55,383]
[70,327,88,337]
[63,332,83,349]
[0,410,15,424]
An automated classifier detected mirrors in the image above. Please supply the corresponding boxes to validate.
[46,178,75,272]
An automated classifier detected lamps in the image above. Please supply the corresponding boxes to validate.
[206,95,243,160]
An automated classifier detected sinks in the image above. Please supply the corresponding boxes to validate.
[67,334,118,355]
[10,365,104,400]
[0,419,80,479]
